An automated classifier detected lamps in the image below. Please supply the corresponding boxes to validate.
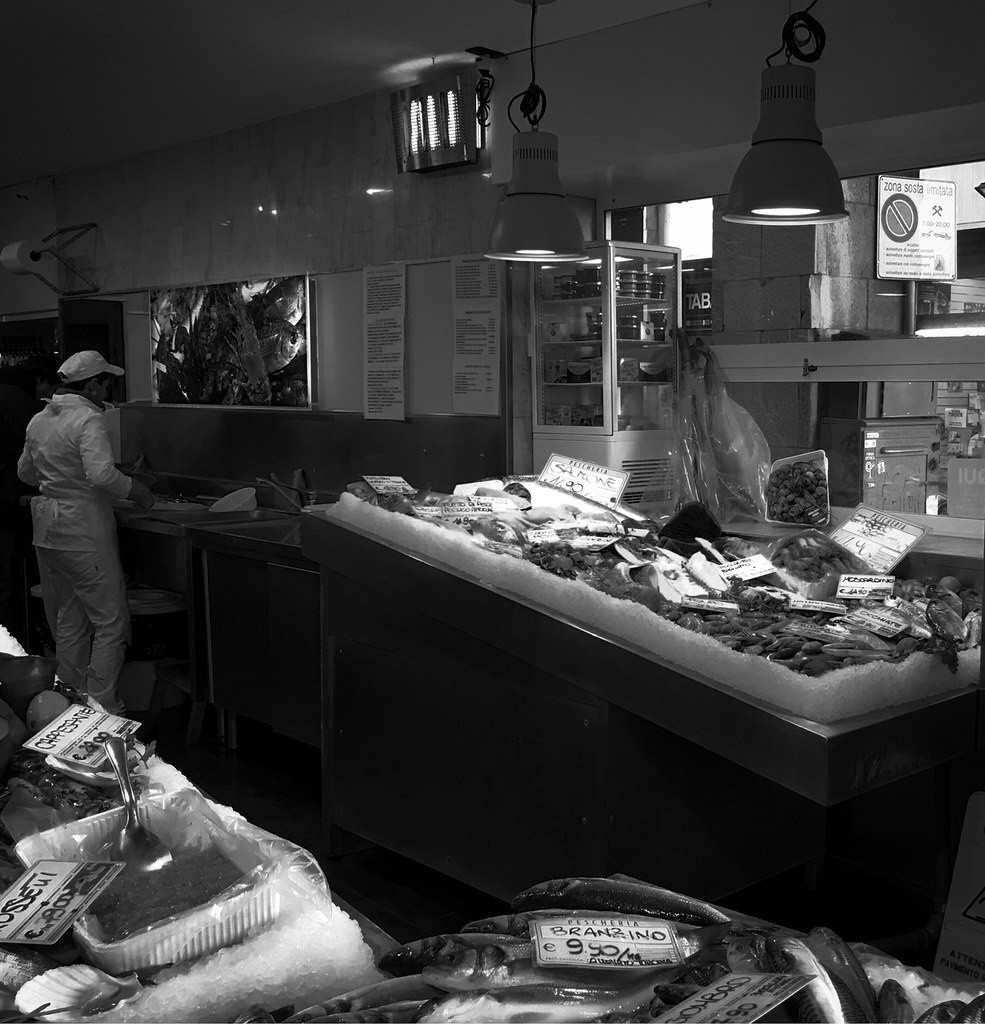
[482,0,587,263]
[719,0,850,227]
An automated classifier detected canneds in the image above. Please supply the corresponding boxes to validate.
[552,265,667,339]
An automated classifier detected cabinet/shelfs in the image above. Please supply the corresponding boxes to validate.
[200,545,322,777]
[17,510,203,744]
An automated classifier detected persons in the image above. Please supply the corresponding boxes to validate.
[17,349,154,720]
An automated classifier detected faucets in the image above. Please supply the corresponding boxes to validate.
[258,473,321,513]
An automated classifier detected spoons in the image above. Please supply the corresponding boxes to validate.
[105,738,174,874]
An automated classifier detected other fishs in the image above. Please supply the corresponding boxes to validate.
[859,574,984,651]
[234,873,985,1023]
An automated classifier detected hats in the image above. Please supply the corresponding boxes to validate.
[58,349,125,382]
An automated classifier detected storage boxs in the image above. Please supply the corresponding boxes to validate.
[712,179,906,343]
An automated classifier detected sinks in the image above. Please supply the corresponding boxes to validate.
[204,515,302,550]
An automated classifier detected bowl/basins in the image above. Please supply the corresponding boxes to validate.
[209,487,257,512]
[124,473,156,485]
[586,312,602,338]
[619,316,637,325]
[619,325,639,339]
[619,269,665,299]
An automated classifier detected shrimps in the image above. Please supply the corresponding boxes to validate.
[772,534,862,581]
